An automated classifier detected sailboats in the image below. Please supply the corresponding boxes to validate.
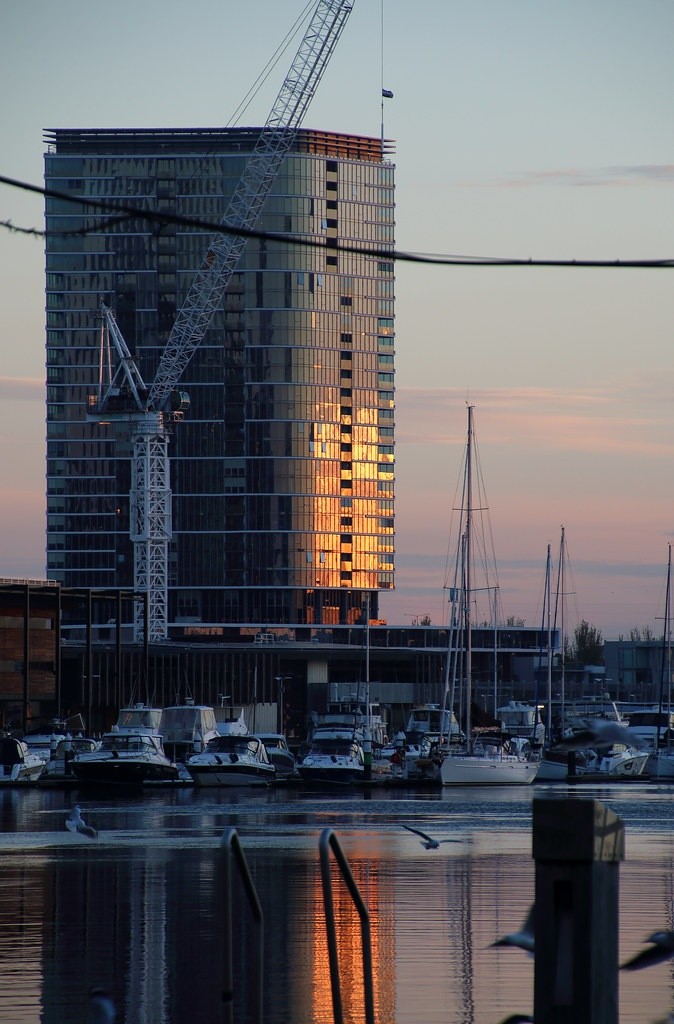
[294,403,674,784]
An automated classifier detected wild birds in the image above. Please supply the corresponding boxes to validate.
[400,825,466,850]
[65,805,98,838]
[619,930,674,973]
[479,903,536,958]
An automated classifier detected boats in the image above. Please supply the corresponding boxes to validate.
[0,697,298,787]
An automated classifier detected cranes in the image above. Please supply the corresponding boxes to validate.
[87,2,354,643]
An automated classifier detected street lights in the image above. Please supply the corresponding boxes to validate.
[404,613,431,626]
